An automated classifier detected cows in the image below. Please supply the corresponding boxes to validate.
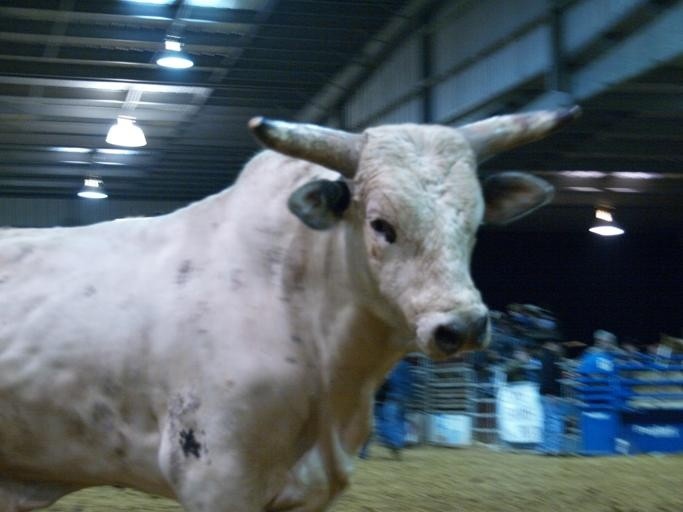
[0,106,584,512]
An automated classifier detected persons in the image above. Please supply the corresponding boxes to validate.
[366,302,682,461]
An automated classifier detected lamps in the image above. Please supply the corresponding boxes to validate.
[77,156,109,200]
[154,30,194,70]
[588,189,625,238]
[105,113,146,149]
